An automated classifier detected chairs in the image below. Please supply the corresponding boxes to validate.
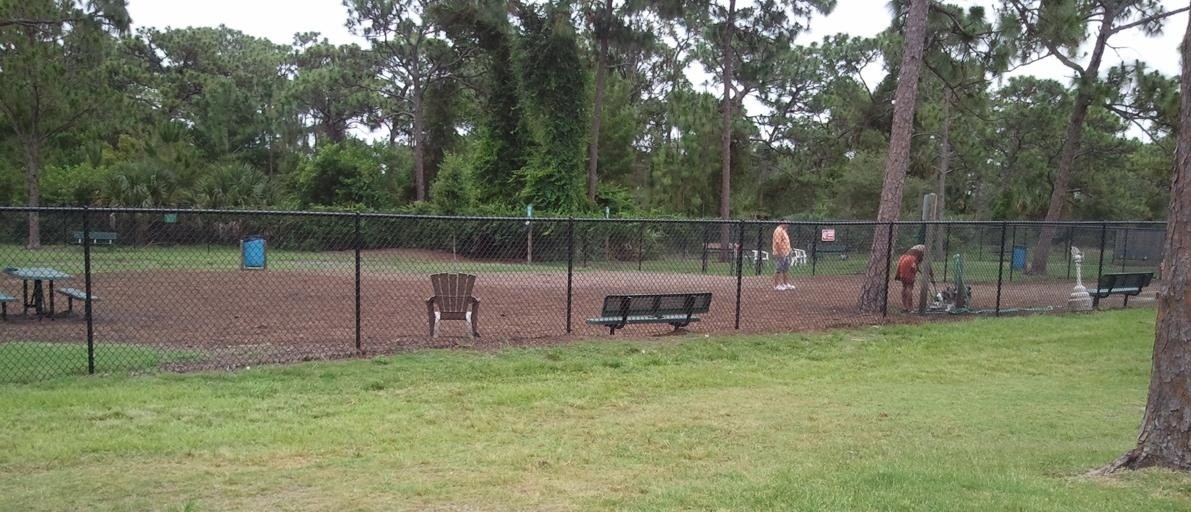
[426,269,483,344]
[750,246,807,269]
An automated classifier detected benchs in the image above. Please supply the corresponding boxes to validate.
[586,290,715,337]
[0,293,17,321]
[70,231,116,246]
[1088,270,1155,309]
[54,286,101,317]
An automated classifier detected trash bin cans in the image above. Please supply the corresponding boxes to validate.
[244,235,263,267]
[1013,245,1027,268]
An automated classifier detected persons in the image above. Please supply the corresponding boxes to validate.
[769,218,796,292]
[898,243,936,314]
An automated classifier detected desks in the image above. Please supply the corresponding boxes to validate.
[3,263,73,321]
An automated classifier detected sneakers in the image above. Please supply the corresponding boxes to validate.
[772,283,787,291]
[786,284,795,291]
